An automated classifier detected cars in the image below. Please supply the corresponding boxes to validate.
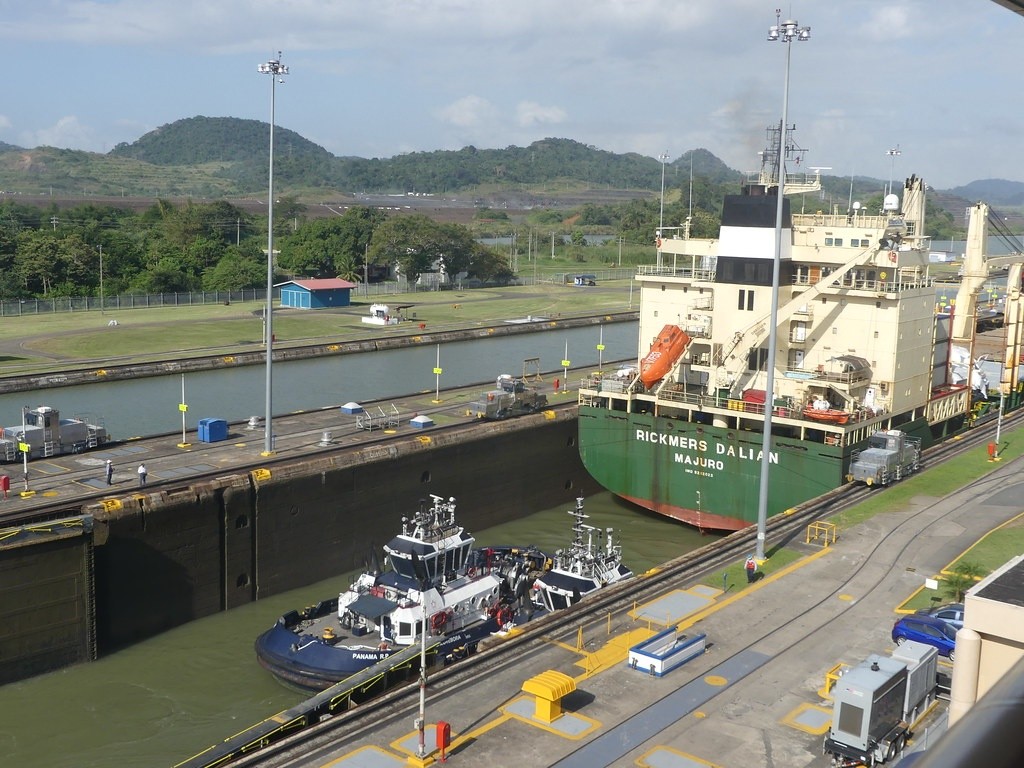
[914,602,965,630]
[891,614,960,662]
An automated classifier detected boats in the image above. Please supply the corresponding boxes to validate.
[576,121,1024,532]
[254,491,556,700]
[440,496,633,670]
[0,406,107,464]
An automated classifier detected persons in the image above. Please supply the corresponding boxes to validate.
[812,396,825,410]
[823,394,831,411]
[383,314,389,326]
[106,459,116,486]
[808,400,814,411]
[138,463,148,487]
[377,638,392,652]
[743,554,758,583]
[856,403,863,419]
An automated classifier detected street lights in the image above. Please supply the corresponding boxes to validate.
[256,52,289,455]
[752,20,814,560]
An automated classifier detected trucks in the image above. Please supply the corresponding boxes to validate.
[821,639,942,768]
